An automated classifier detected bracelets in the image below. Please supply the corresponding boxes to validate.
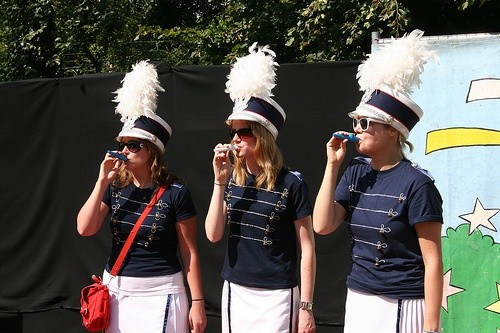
[192,299,204,301]
[214,182,227,186]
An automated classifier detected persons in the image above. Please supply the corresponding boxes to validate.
[312,81,443,333]
[76,112,207,333]
[203,94,316,333]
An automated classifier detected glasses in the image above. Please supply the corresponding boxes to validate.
[118,140,150,155]
[352,118,386,131]
[229,127,255,139]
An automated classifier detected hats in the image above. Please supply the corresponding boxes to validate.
[346,29,439,142]
[224,42,287,141]
[110,59,172,152]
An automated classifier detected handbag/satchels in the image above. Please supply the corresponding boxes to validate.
[77,275,112,331]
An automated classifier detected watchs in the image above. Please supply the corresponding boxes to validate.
[299,300,314,311]
